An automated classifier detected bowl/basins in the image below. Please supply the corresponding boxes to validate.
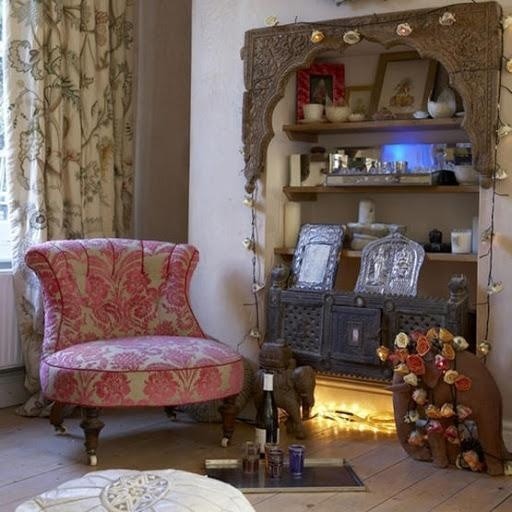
[454,166,479,187]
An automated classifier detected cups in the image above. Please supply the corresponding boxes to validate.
[289,446,306,478]
[302,105,323,120]
[325,106,351,124]
[451,223,474,254]
[268,449,285,479]
[432,171,455,187]
[378,163,392,175]
[264,443,282,474]
[396,162,409,175]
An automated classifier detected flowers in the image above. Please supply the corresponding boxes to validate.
[239,1,512,397]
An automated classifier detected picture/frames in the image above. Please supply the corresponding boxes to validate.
[367,51,437,119]
[297,60,345,125]
[346,86,372,115]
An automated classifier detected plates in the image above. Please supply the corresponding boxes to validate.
[297,119,329,123]
[206,458,367,492]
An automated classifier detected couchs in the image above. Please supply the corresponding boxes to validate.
[25,238,244,466]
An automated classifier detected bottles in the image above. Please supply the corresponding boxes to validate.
[371,161,375,170]
[255,368,280,460]
[348,158,367,175]
[339,159,343,172]
[429,223,443,252]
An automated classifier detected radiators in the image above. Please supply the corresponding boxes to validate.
[0,269,25,372]
